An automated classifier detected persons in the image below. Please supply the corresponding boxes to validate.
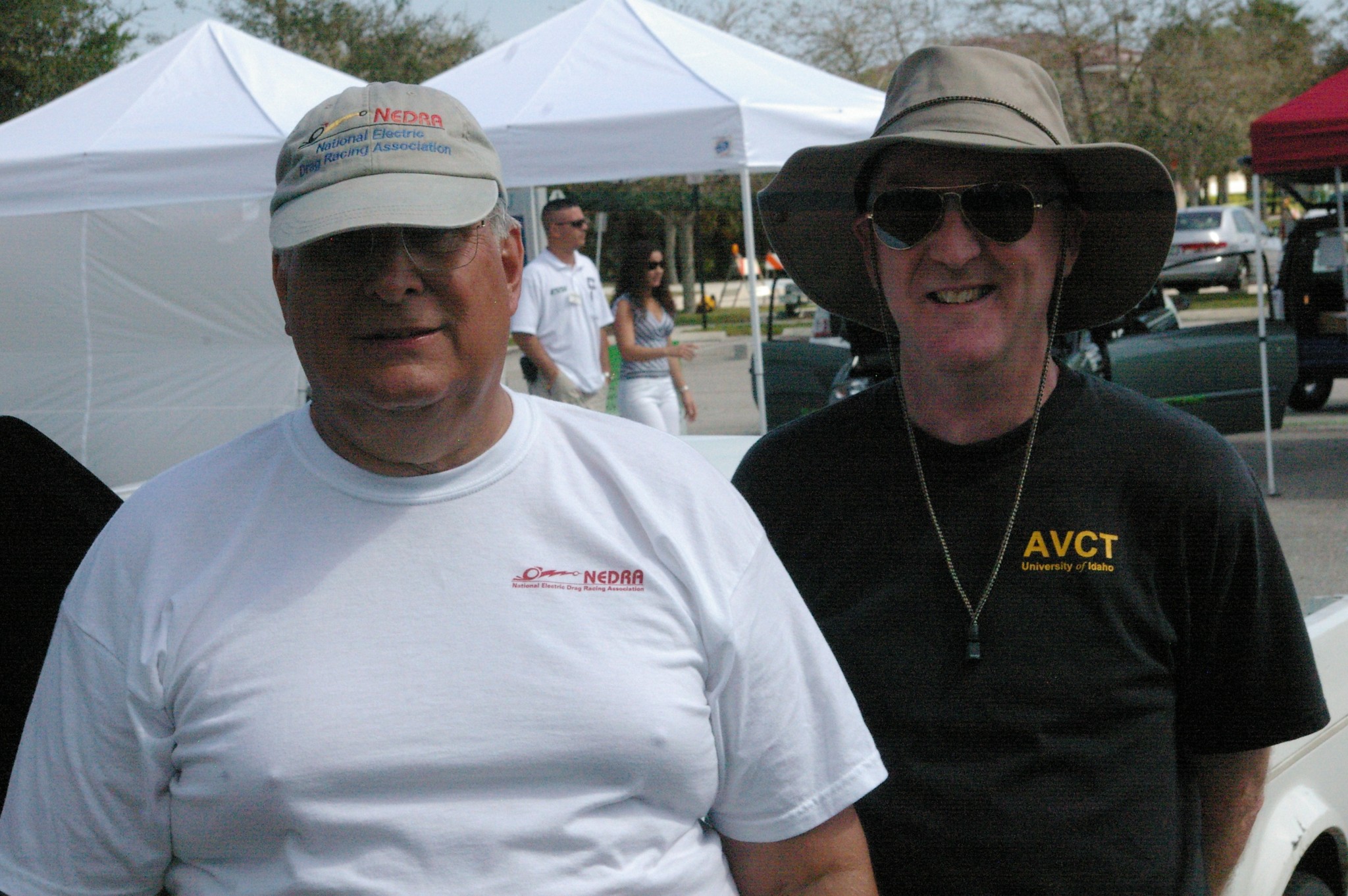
[0,80,891,896]
[609,237,698,436]
[730,45,1331,896]
[695,294,719,313]
[509,198,616,413]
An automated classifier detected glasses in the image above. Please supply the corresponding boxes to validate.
[297,211,501,278]
[645,261,666,270]
[553,217,589,229]
[853,180,1058,252]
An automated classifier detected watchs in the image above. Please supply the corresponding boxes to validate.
[602,372,615,382]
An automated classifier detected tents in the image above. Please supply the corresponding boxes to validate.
[0,17,370,502]
[1248,67,1348,498]
[419,0,886,436]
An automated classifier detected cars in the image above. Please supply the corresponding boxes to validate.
[748,267,1300,443]
[1236,151,1348,416]
[1158,204,1285,298]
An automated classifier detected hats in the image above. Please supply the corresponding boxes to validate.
[268,82,508,249]
[757,47,1177,335]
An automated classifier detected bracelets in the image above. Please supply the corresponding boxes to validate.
[678,385,690,393]
